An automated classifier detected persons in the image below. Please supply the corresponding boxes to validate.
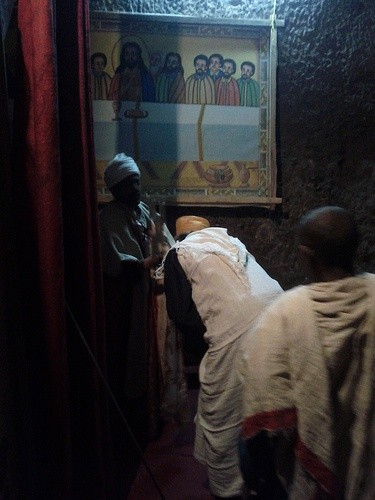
[240,206,375,500]
[163,215,283,500]
[97,151,176,437]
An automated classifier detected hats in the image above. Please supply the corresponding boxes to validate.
[176,216,211,236]
[103,153,142,191]
